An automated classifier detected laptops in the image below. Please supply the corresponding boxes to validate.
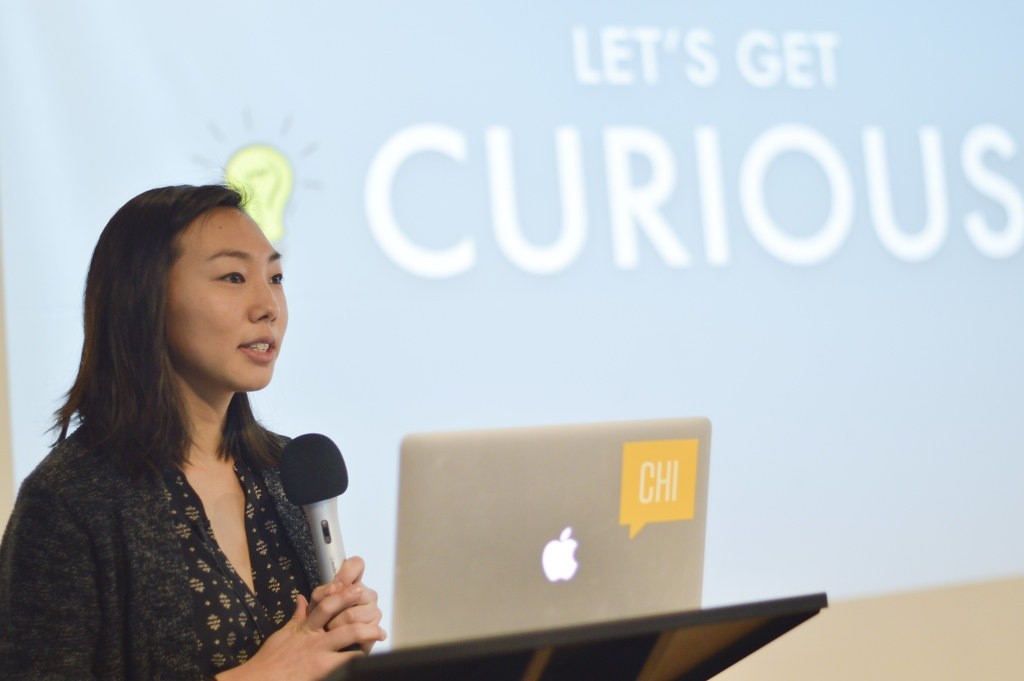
[389,416,713,651]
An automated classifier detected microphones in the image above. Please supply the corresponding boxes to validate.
[279,433,353,617]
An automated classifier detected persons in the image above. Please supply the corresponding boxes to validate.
[0,177,389,680]
